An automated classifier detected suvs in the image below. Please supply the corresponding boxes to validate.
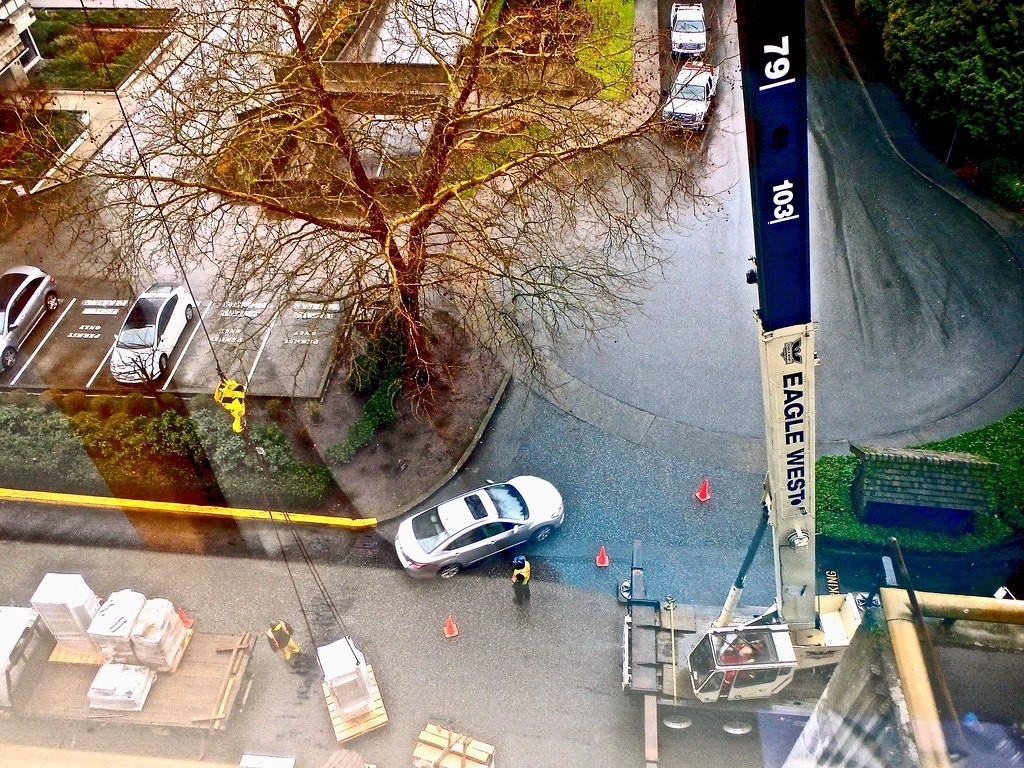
[0,266,58,372]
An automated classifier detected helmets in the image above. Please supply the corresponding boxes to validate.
[513,555,525,564]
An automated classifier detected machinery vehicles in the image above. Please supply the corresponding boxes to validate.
[79,0,862,740]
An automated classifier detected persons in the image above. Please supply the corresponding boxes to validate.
[512,556,531,605]
[267,619,302,663]
[717,644,754,693]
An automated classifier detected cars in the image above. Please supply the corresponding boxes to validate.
[109,282,194,383]
[395,475,564,580]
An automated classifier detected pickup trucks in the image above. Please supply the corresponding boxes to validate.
[661,62,721,132]
[668,3,706,58]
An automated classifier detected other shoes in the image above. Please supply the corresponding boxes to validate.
[513,596,522,604]
[525,596,530,600]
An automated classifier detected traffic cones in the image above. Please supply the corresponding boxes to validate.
[596,545,609,567]
[695,479,711,501]
[443,615,458,638]
